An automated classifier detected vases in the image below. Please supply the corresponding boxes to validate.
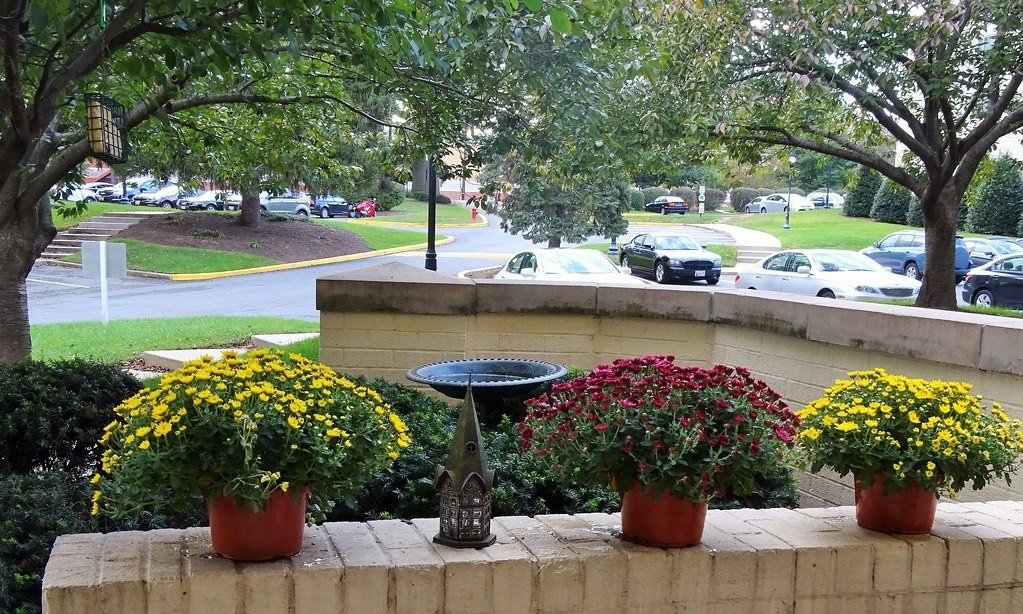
[621,479,709,548]
[210,482,308,562]
[854,474,945,534]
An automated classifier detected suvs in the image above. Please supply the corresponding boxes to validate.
[859,230,974,286]
[224,185,311,219]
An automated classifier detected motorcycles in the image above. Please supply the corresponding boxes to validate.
[349,197,377,218]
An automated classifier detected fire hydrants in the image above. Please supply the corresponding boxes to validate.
[471,207,479,221]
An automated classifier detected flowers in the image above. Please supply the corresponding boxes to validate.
[516,355,802,508]
[90,347,415,518]
[795,367,1023,501]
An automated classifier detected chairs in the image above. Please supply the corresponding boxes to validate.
[1003,262,1013,270]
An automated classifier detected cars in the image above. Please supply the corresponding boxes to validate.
[963,237,1023,274]
[306,192,355,218]
[619,232,722,285]
[734,248,922,301]
[810,193,844,210]
[49,181,114,203]
[760,194,816,213]
[119,178,181,208]
[494,249,646,285]
[962,252,1023,310]
[98,182,141,204]
[745,196,764,214]
[176,190,234,211]
[131,185,205,208]
[645,195,688,215]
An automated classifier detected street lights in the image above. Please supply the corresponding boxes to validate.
[783,150,797,229]
